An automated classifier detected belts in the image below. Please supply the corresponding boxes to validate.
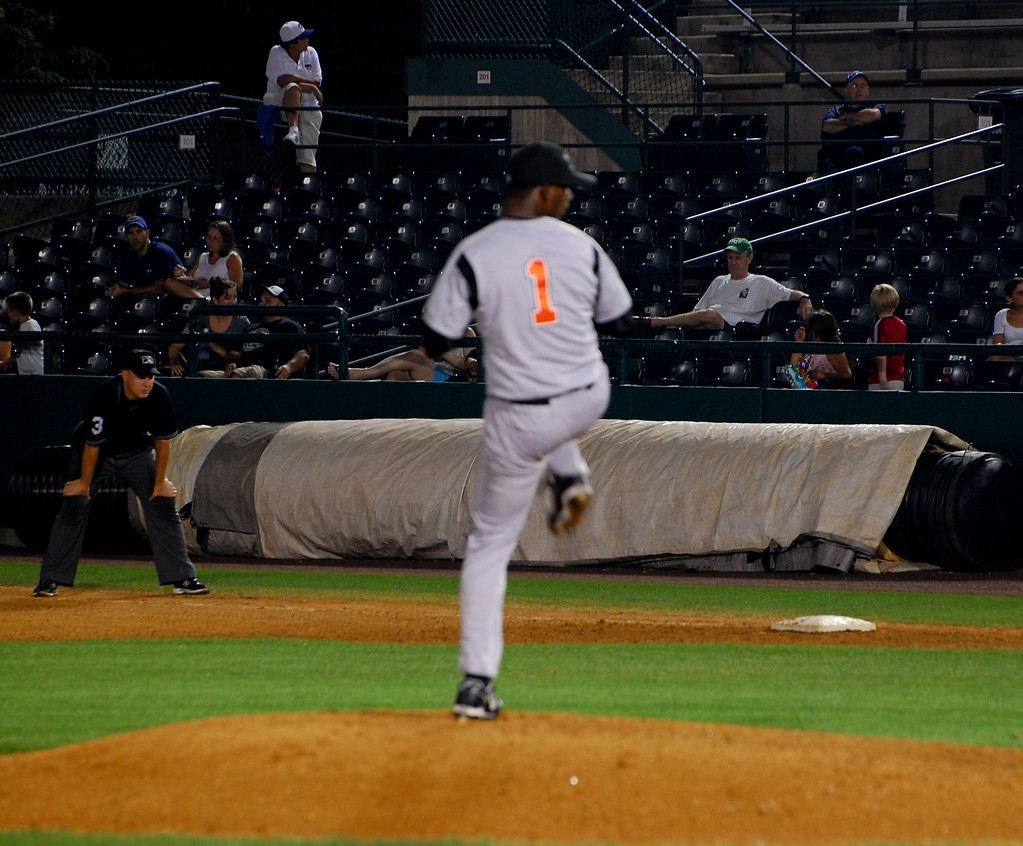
[512,383,593,405]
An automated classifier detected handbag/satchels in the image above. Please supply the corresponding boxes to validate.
[786,363,819,389]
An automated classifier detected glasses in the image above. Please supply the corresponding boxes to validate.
[205,235,225,244]
[847,82,868,90]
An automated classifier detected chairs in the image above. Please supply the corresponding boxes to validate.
[0,112,1023,390]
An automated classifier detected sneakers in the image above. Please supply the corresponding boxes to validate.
[548,475,593,535]
[284,128,301,145]
[453,676,501,717]
[34,579,58,596]
[173,577,208,595]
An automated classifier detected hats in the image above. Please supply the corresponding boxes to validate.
[844,70,869,84]
[262,284,289,305]
[500,142,597,190]
[723,237,752,253]
[281,21,314,43]
[119,349,160,376]
[125,216,147,230]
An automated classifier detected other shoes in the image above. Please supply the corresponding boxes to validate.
[325,364,341,379]
[633,316,656,330]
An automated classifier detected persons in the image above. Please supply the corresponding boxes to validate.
[991,277,1023,362]
[166,220,243,304]
[790,310,852,381]
[868,284,907,390]
[0,291,44,375]
[822,70,887,169]
[168,276,310,379]
[105,216,183,298]
[632,237,812,342]
[263,20,323,172]
[32,349,209,596]
[421,140,633,718]
[327,326,485,380]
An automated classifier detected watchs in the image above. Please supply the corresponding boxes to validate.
[799,294,809,302]
